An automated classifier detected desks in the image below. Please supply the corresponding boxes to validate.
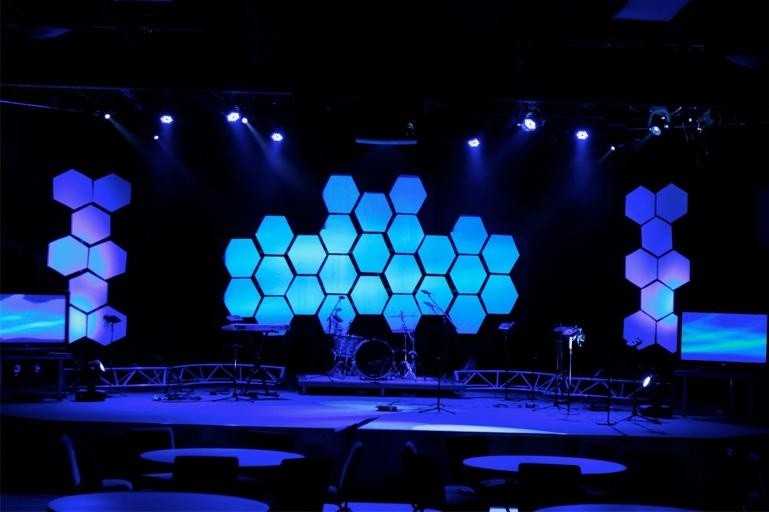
[48,492,270,511]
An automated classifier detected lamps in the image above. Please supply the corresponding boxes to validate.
[642,370,673,417]
[648,112,669,136]
[223,104,240,122]
[517,105,546,132]
[66,359,106,401]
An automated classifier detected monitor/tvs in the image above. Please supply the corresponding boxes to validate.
[0,292,69,345]
[676,310,768,366]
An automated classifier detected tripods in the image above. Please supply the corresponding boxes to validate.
[618,384,663,425]
[419,377,456,415]
[532,335,580,412]
[328,319,418,382]
[212,350,255,403]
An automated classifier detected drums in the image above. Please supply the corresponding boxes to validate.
[335,333,364,360]
[353,336,395,381]
